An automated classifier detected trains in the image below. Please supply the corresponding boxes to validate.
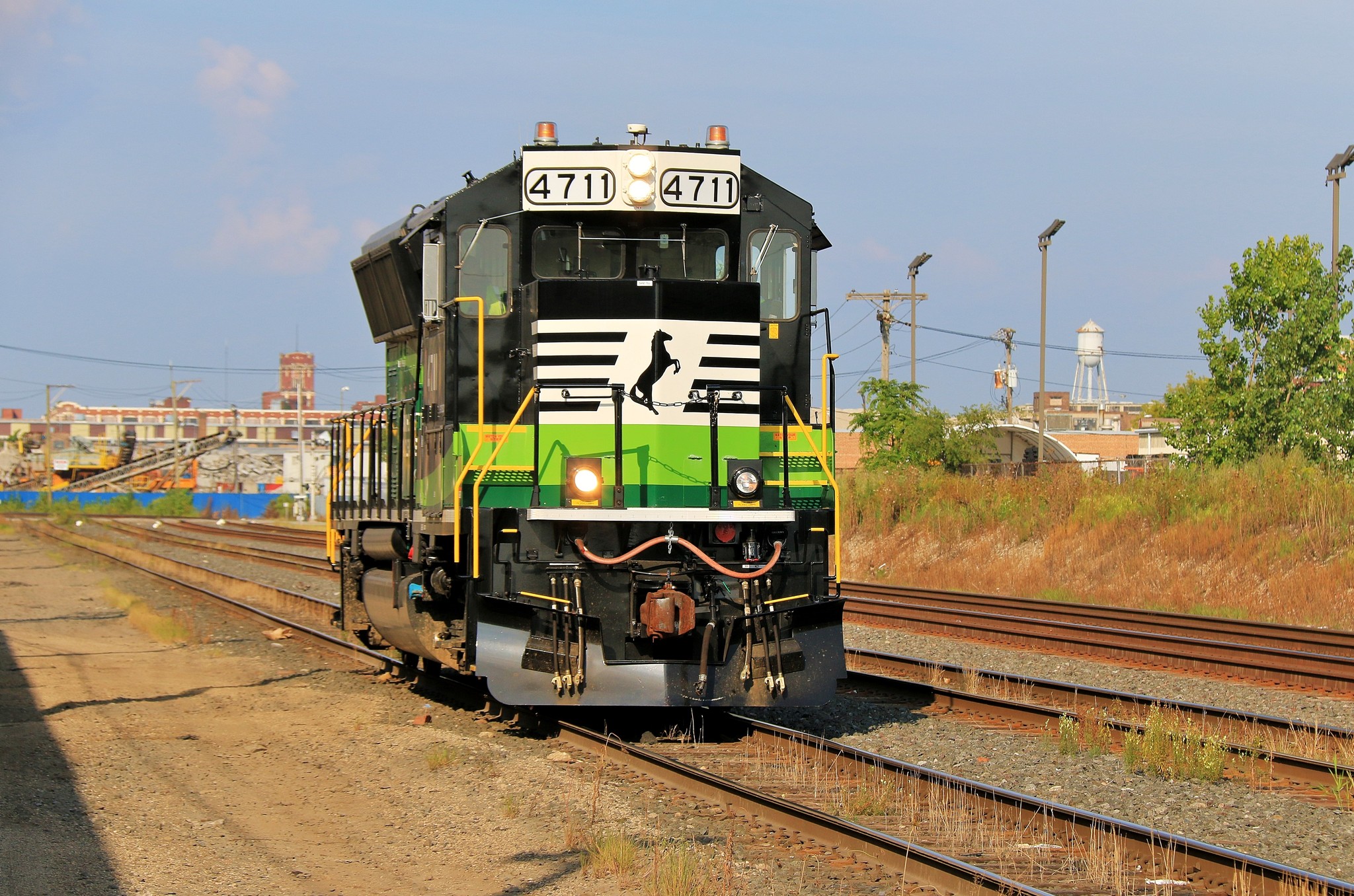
[325,121,851,734]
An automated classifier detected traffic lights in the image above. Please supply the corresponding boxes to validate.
[1022,447,1039,463]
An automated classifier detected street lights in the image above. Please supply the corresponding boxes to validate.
[1325,142,1354,323]
[907,251,934,414]
[1037,218,1067,471]
